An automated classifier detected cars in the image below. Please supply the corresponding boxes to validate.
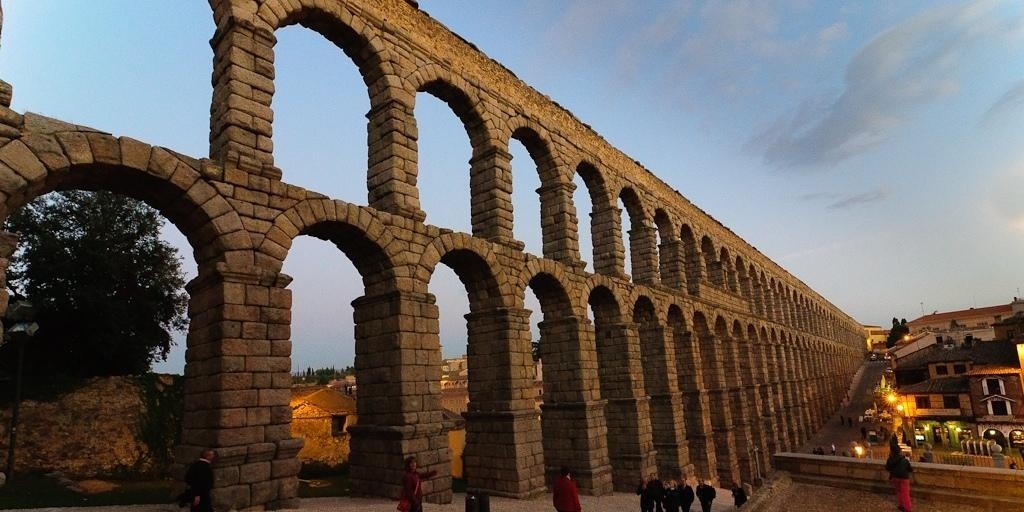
[870,354,877,361]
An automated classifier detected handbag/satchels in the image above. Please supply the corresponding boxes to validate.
[398,499,413,511]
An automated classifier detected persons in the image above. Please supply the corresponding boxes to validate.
[1010,460,1018,469]
[553,467,581,512]
[731,483,747,508]
[885,445,913,512]
[636,473,716,512]
[176,448,216,512]
[397,456,423,512]
[813,415,866,458]
